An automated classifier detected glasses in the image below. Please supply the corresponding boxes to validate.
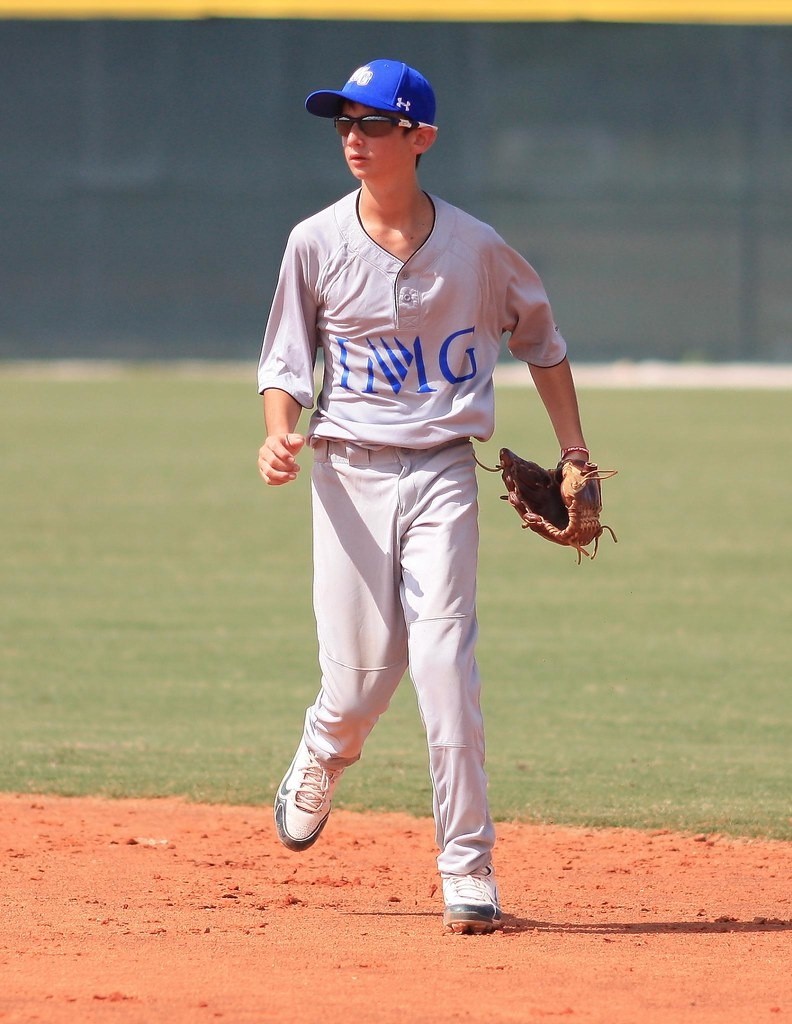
[333,114,438,138]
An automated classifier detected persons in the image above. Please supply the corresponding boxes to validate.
[258,59,602,930]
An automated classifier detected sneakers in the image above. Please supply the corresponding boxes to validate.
[442,861,502,935]
[273,733,344,852]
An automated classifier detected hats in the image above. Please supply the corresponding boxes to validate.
[304,58,436,126]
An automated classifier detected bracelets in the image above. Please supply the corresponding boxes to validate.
[562,446,590,460]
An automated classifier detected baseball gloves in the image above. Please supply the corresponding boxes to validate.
[499,446,604,547]
